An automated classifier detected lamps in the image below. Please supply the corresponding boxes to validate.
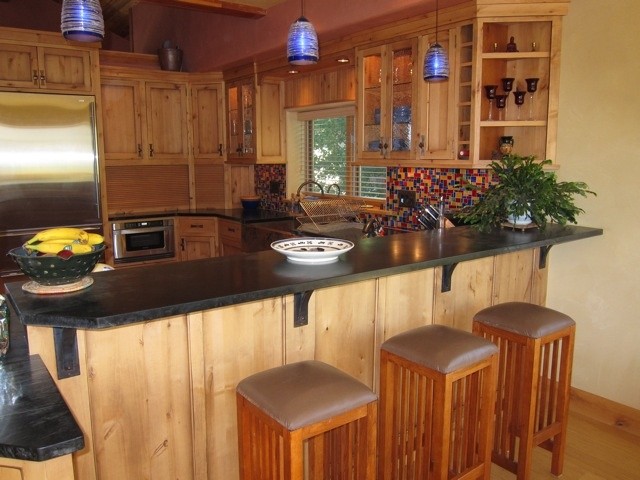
[60,0,108,46]
[422,0,450,83]
[283,0,321,67]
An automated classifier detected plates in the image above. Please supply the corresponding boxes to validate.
[269,235,356,265]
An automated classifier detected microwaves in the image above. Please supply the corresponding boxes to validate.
[111,217,178,265]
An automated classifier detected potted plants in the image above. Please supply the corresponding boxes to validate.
[448,155,599,232]
[159,37,185,70]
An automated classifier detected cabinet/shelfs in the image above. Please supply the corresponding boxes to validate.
[225,72,256,163]
[0,26,101,96]
[99,64,188,165]
[359,37,421,166]
[175,216,218,261]
[450,10,560,171]
[189,78,229,160]
[420,30,456,162]
[213,218,242,256]
[254,77,283,164]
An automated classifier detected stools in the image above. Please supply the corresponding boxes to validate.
[473,300,576,479]
[233,358,379,479]
[380,320,500,479]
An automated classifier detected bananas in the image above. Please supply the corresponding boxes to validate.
[22,227,105,255]
